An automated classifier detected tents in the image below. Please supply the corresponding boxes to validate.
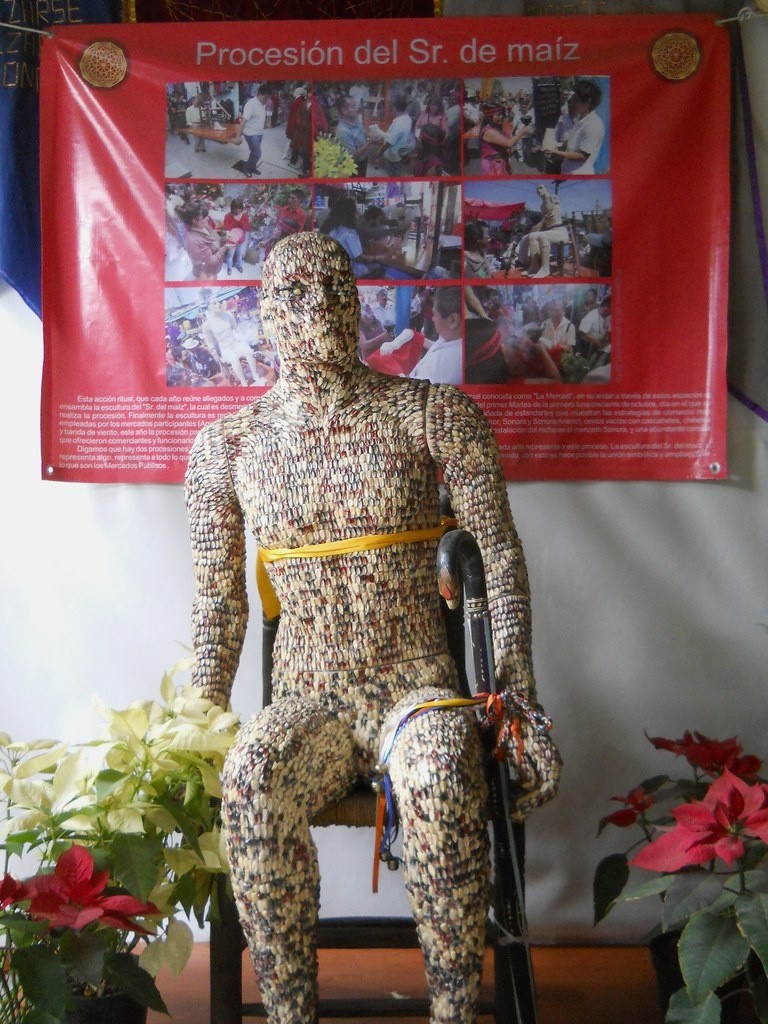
[463,195,526,223]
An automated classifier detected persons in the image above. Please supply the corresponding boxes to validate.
[520,181,570,280]
[351,284,462,386]
[168,79,312,177]
[485,212,525,269]
[466,79,606,177]
[163,180,306,279]
[165,286,281,388]
[312,80,461,177]
[182,232,564,1023]
[459,219,495,280]
[314,188,434,278]
[578,211,613,277]
[462,284,611,383]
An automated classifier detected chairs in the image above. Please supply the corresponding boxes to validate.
[210,482,531,1024]
[555,222,582,277]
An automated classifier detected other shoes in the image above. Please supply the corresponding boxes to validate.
[228,269,231,275]
[254,170,261,175]
[245,170,252,178]
[238,267,244,273]
[518,157,524,162]
[528,158,533,163]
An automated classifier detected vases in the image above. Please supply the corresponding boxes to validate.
[648,932,743,1024]
[60,954,154,1024]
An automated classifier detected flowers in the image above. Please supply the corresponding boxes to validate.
[0,669,246,1024]
[314,131,359,178]
[588,732,768,1024]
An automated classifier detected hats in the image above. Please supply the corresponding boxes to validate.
[182,338,199,349]
